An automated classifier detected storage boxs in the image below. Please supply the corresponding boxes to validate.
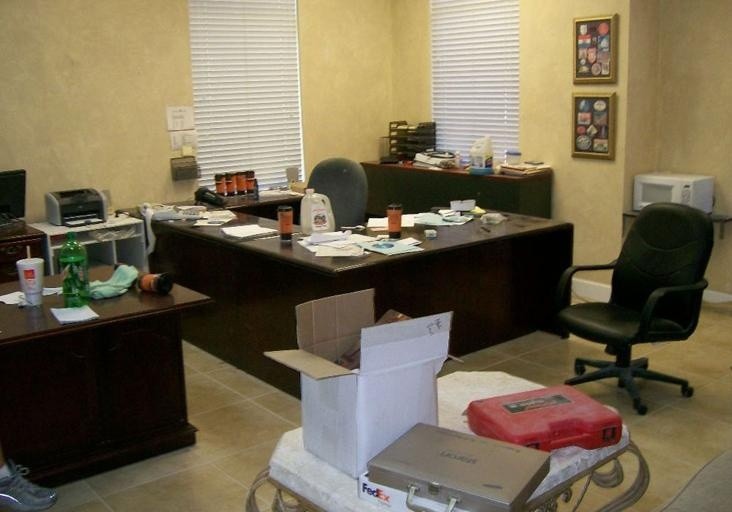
[261,287,463,480]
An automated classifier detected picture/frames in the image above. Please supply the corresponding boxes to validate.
[570,13,617,161]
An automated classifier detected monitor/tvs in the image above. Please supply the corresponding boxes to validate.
[0,168,27,224]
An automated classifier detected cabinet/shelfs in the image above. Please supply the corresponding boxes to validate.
[359,159,552,221]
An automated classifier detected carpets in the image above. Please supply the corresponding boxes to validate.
[660,447,732,511]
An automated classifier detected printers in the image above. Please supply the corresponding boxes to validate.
[44,187,108,228]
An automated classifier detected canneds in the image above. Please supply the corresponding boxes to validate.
[139,274,174,294]
[247,171,255,193]
[214,173,225,195]
[278,207,294,241]
[506,151,522,164]
[236,173,246,194]
[226,173,236,195]
[387,203,403,240]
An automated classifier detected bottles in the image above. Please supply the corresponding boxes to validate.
[59,232,88,303]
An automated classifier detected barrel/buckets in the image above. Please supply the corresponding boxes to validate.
[301,189,335,236]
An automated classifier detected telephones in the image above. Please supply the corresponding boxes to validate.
[195,187,224,207]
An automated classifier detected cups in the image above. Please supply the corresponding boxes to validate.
[213,170,258,195]
[276,209,293,244]
[389,206,403,237]
[17,259,45,307]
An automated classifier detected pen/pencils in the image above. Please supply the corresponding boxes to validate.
[481,226,491,232]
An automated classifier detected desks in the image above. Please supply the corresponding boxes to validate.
[115,184,573,400]
[27,211,148,275]
[244,370,651,511]
[1,265,215,489]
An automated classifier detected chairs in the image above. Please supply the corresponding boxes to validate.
[307,158,369,231]
[552,202,714,417]
